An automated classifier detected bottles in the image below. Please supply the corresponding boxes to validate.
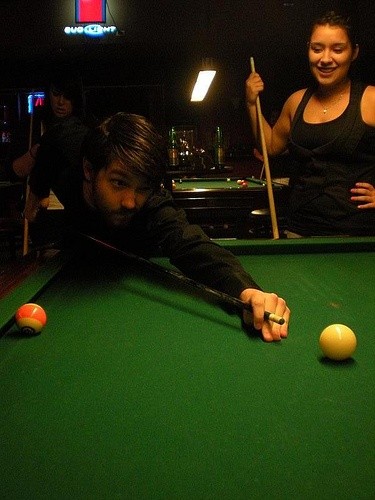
[168,122,177,164]
[215,125,225,170]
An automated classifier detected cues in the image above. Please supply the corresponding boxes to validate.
[22,205,285,325]
[249,55,279,238]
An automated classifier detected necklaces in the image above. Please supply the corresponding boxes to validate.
[316,86,346,114]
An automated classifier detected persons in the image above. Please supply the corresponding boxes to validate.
[245,10,375,237]
[22,113,290,343]
[9,60,84,259]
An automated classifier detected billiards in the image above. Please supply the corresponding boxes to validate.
[237,180,247,186]
[15,303,46,335]
[227,178,231,182]
[171,179,176,189]
[179,179,182,183]
[318,322,358,362]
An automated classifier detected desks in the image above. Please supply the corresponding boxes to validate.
[171,176,285,239]
[1,236,375,500]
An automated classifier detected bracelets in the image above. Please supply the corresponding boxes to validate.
[29,150,35,159]
